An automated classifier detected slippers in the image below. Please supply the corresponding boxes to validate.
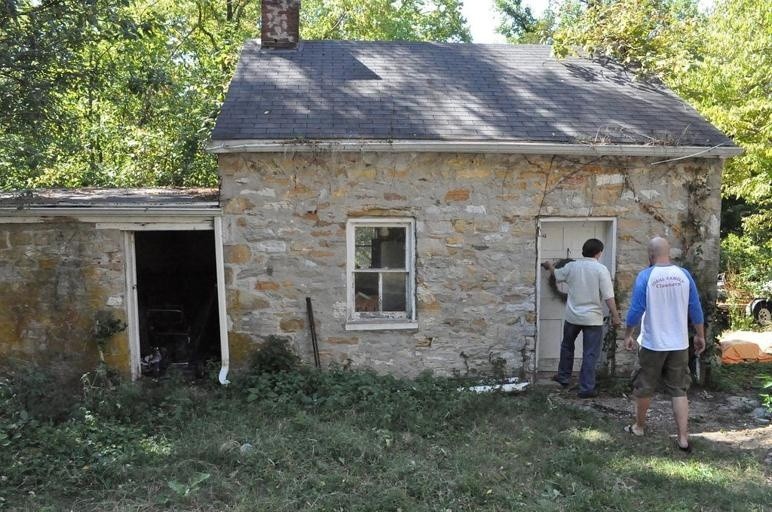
[674,438,690,451]
[623,424,644,439]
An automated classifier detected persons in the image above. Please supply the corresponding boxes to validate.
[542,239,622,399]
[624,236,706,449]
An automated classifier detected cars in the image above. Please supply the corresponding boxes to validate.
[716,264,772,328]
